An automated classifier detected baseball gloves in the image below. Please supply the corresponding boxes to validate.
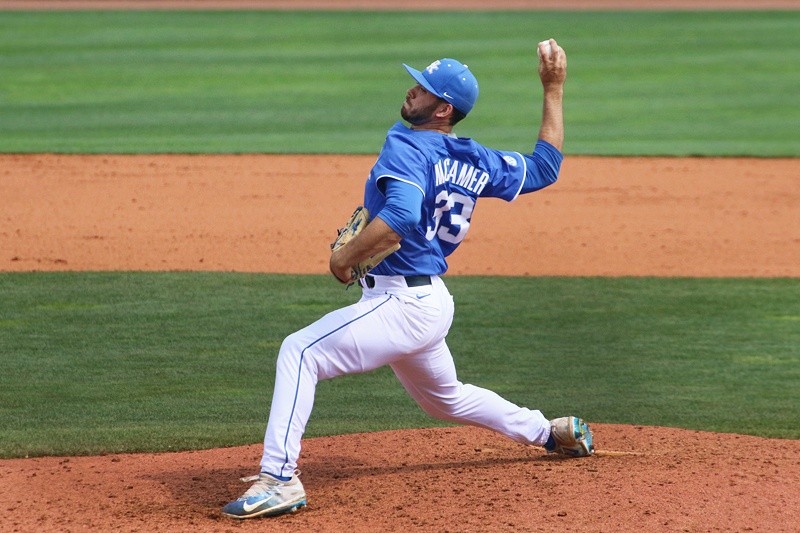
[330,205,401,285]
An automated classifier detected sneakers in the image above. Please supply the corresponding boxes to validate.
[222,469,307,519]
[547,416,595,457]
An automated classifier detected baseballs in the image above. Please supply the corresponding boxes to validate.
[536,40,558,60]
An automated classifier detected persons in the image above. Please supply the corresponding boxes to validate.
[220,37,595,520]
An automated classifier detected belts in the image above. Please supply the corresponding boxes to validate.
[358,273,432,288]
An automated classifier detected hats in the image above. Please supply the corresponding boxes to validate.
[402,58,479,115]
